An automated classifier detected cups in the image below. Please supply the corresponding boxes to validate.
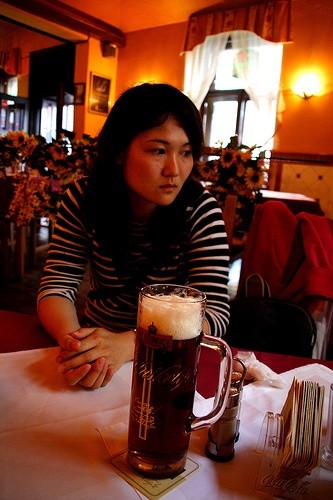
[126,285,233,480]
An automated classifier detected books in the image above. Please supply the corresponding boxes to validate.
[280,377,325,483]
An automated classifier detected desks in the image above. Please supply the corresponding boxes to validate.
[0,310,333,500]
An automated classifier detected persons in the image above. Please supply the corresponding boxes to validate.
[36,83,231,389]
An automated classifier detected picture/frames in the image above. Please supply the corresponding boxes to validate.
[88,71,113,116]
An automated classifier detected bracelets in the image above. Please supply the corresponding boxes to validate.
[132,328,138,337]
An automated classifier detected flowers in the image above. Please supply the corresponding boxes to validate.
[0,129,97,226]
[196,140,271,197]
